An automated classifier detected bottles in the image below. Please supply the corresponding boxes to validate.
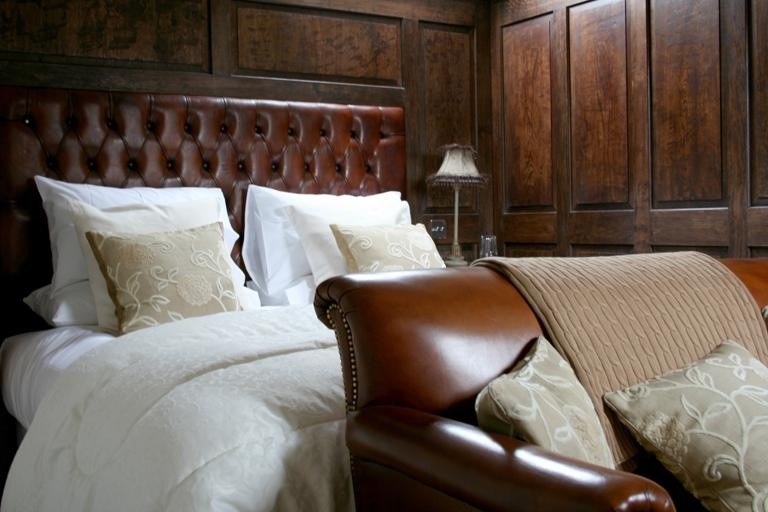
[479,234,498,258]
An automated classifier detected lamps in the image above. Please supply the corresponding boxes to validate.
[420,140,490,270]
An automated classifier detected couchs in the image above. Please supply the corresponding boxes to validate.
[305,239,768,510]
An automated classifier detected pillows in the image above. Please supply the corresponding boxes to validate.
[462,324,623,473]
[23,280,261,328]
[32,174,224,297]
[234,180,450,313]
[594,335,768,511]
[65,198,221,334]
[85,221,243,333]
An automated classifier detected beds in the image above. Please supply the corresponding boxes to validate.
[1,77,459,511]
